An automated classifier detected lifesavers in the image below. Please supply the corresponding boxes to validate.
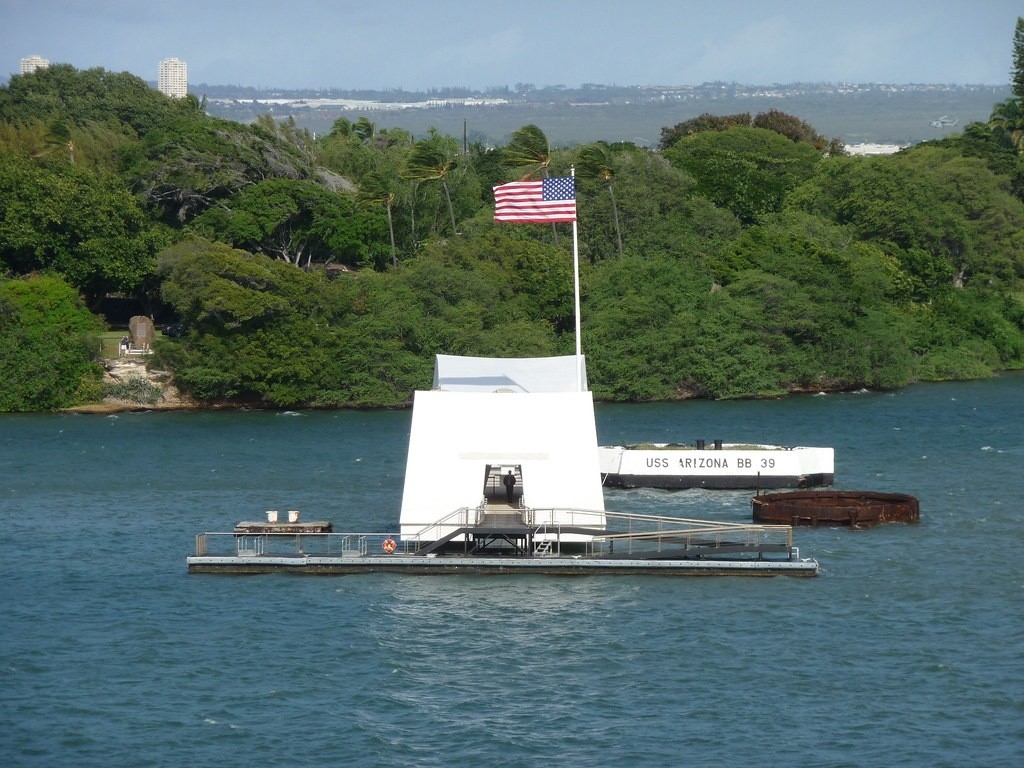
[382,538,396,553]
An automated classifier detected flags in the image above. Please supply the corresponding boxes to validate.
[492,176,577,223]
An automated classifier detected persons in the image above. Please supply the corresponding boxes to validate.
[504,471,516,503]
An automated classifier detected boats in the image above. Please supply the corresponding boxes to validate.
[596,440,836,490]
